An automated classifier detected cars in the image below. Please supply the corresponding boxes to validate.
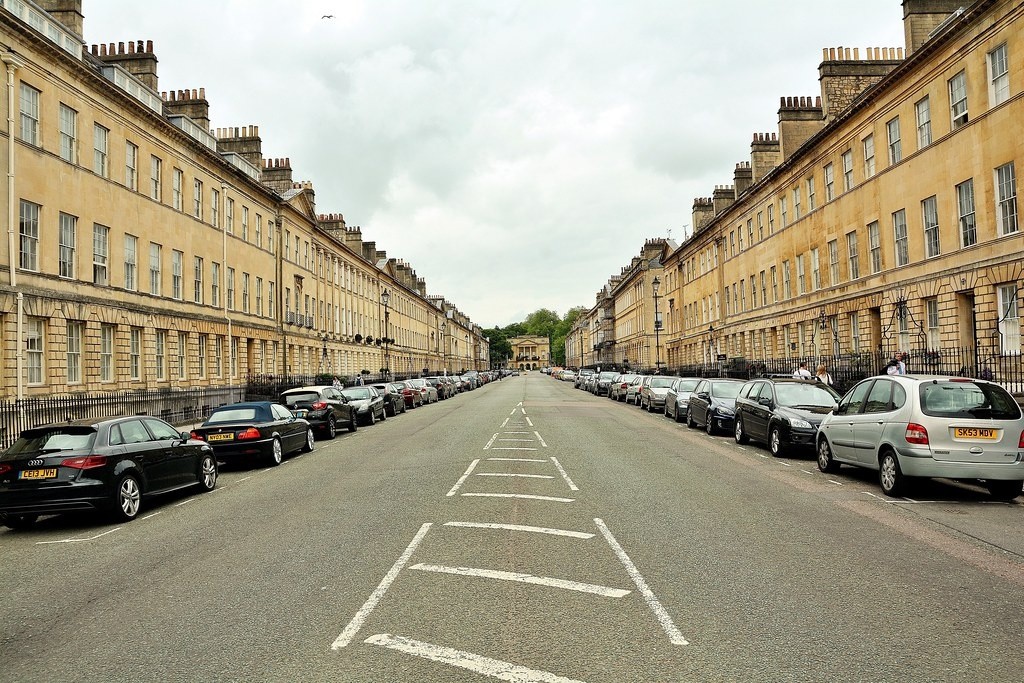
[686,378,750,436]
[733,375,843,457]
[626,376,654,406]
[190,402,316,466]
[392,381,423,409]
[664,377,708,422]
[814,370,1023,496]
[340,386,387,425]
[641,376,682,411]
[540,365,576,381]
[424,368,520,401]
[407,378,439,403]
[366,383,407,416]
[0,415,219,530]
[580,371,638,401]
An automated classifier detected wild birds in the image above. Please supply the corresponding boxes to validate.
[322,15,336,19]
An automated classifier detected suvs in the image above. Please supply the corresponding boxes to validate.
[574,368,595,388]
[279,385,358,440]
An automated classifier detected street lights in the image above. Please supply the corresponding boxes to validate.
[579,318,600,372]
[380,287,481,382]
[651,276,661,374]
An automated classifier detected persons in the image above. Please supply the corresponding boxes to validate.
[880,354,905,375]
[499,370,503,381]
[654,368,660,375]
[620,368,627,374]
[792,362,812,380]
[333,376,341,389]
[356,374,364,386]
[815,365,834,386]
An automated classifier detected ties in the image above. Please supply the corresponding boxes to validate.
[898,365,903,374]
